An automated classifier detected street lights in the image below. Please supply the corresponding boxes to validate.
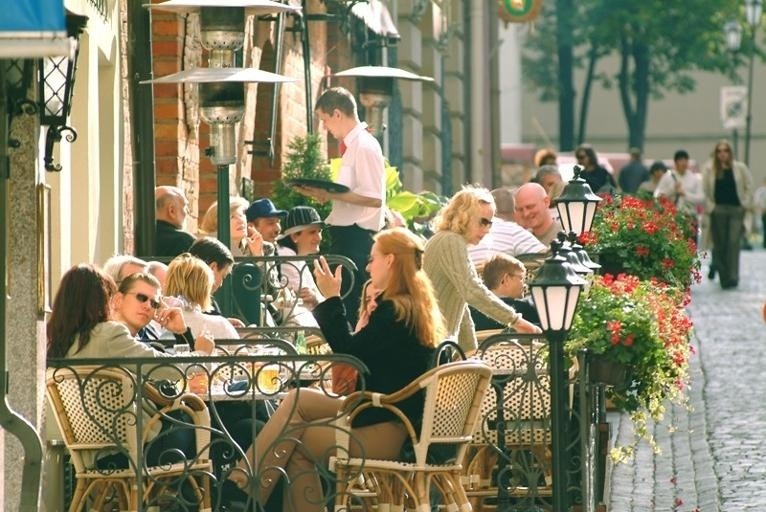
[139,0,304,251]
[333,62,437,151]
[725,0,760,165]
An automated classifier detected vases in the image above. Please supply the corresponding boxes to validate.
[589,356,629,387]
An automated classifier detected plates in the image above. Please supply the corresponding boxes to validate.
[282,177,348,194]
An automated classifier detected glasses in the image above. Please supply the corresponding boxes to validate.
[124,292,161,310]
[479,217,493,230]
[578,155,587,161]
[716,149,730,154]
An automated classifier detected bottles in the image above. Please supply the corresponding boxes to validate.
[296,331,306,355]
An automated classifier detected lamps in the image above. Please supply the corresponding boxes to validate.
[569,232,601,270]
[554,164,604,237]
[558,231,593,299]
[528,239,584,340]
[37,14,89,171]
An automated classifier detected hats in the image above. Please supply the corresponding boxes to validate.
[247,197,289,221]
[274,204,328,243]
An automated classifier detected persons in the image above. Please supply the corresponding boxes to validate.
[576,140,766,290]
[47,149,565,512]
[293,87,385,332]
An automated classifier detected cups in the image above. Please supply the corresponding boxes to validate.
[174,344,190,356]
[188,372,208,397]
[262,364,278,391]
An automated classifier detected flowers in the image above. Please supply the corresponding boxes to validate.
[576,193,702,307]
[543,273,696,466]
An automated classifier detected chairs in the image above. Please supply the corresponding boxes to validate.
[475,263,542,296]
[45,366,214,511]
[334,361,492,512]
[467,329,579,512]
[515,253,548,264]
